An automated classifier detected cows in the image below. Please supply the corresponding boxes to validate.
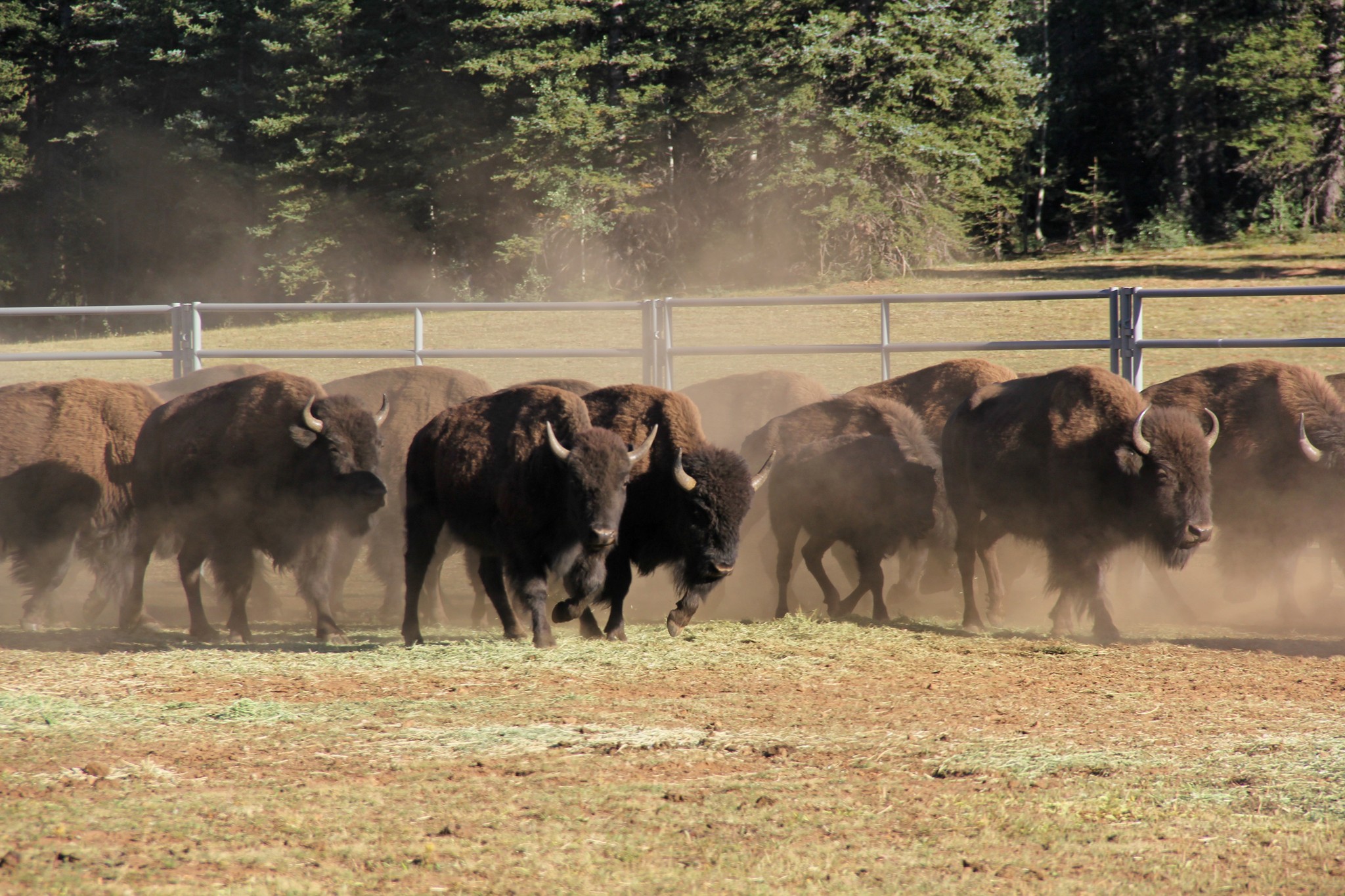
[0,357,1344,655]
